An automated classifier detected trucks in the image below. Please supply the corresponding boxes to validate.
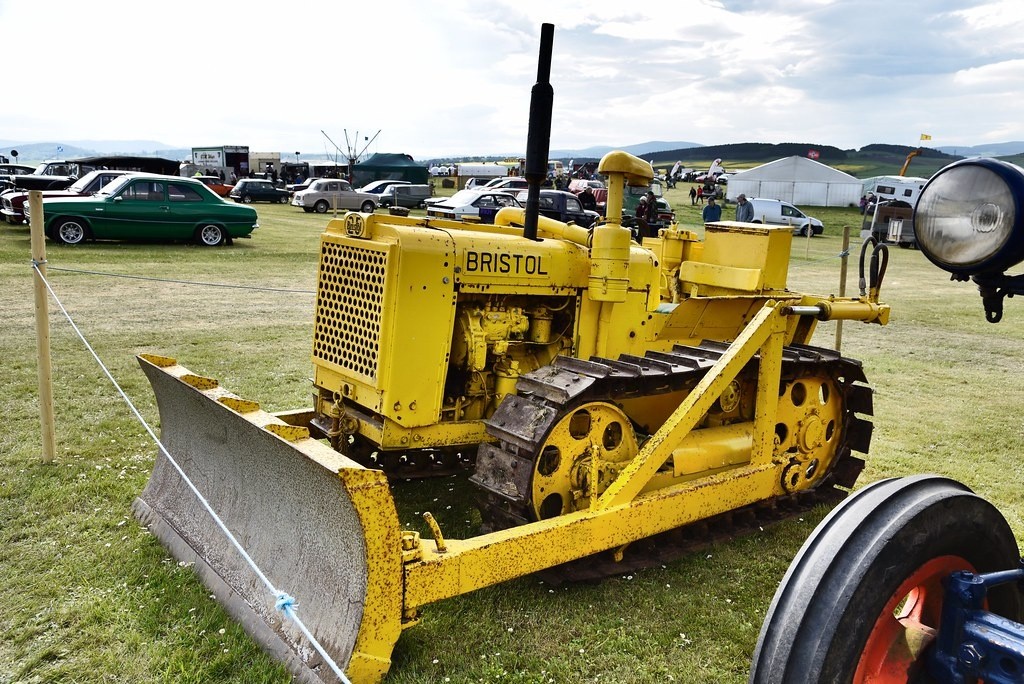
[376,184,432,209]
[745,196,824,237]
[886,216,920,250]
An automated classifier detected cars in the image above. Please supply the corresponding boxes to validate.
[507,188,601,230]
[291,178,379,213]
[286,177,324,197]
[0,161,187,226]
[423,186,545,224]
[464,176,528,190]
[650,168,733,185]
[191,176,235,198]
[703,184,723,199]
[21,174,260,247]
[570,179,605,195]
[230,178,290,205]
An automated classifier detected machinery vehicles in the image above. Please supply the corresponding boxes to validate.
[123,22,891,684]
[748,154,1023,684]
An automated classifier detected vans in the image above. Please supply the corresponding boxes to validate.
[548,160,564,176]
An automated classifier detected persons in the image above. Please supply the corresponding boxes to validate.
[219,170,225,184]
[695,184,703,204]
[666,174,676,189]
[577,186,596,211]
[555,174,572,192]
[271,167,304,184]
[212,168,218,176]
[206,169,212,176]
[249,168,256,178]
[702,196,722,222]
[688,187,696,205]
[195,170,203,176]
[636,191,658,223]
[735,193,754,223]
[229,171,237,186]
[859,195,868,215]
[543,175,554,187]
[263,171,268,179]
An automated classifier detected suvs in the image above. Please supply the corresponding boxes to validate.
[356,180,412,194]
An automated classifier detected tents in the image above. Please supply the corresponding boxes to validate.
[726,155,862,207]
[352,152,429,189]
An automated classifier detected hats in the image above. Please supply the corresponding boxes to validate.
[640,196,647,202]
[735,193,745,198]
